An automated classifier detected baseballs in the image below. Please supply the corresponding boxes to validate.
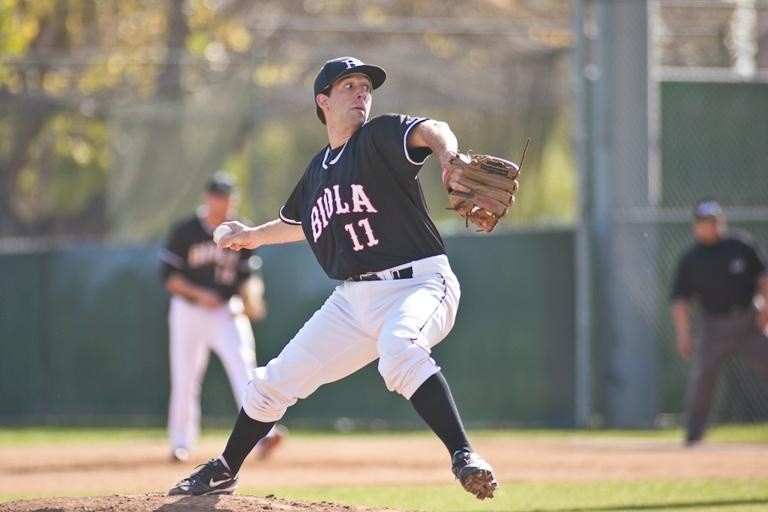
[213,225,232,245]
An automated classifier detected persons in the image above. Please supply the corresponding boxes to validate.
[163,55,520,502]
[662,198,766,449]
[158,172,285,467]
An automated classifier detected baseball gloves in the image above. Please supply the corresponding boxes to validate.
[446,138,530,232]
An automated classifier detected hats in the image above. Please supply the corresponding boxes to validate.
[314,57,386,92]
[204,174,238,194]
[694,200,721,220]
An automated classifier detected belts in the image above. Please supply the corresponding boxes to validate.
[348,267,412,281]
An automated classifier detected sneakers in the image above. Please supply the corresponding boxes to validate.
[451,450,496,500]
[257,433,280,458]
[169,459,239,495]
